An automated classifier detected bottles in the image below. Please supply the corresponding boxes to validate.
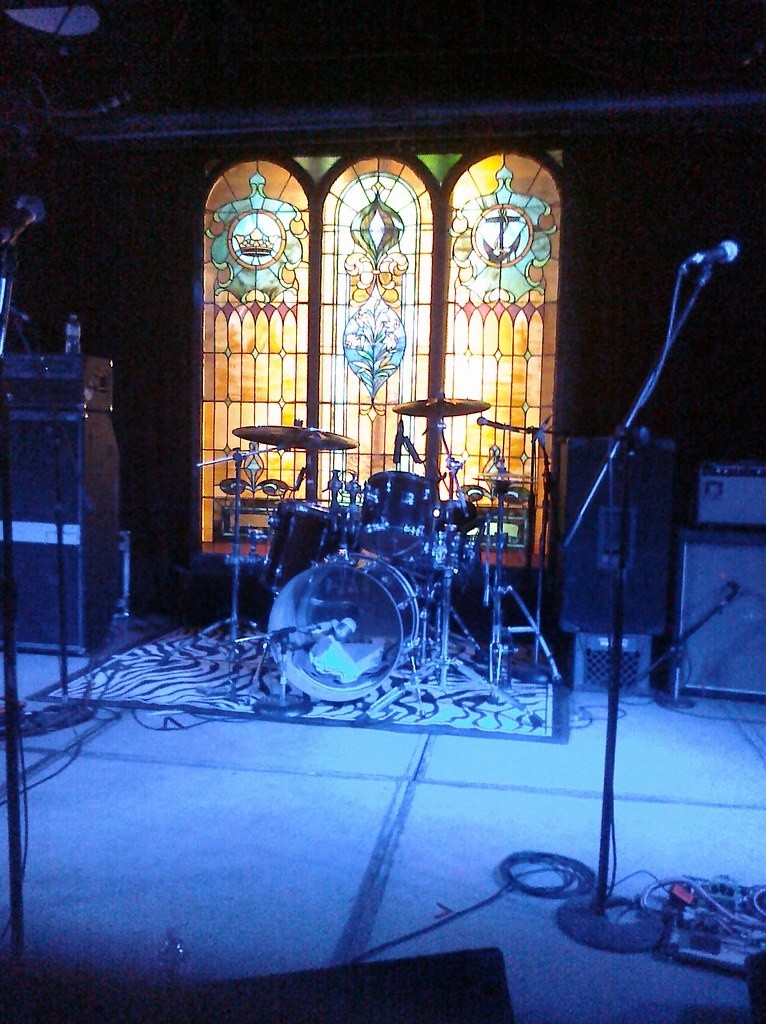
[154,926,189,977]
[65,315,81,353]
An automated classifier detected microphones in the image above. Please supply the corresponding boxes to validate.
[530,417,551,443]
[716,581,738,615]
[476,416,519,433]
[393,420,404,463]
[334,617,357,640]
[680,240,738,270]
[0,194,47,264]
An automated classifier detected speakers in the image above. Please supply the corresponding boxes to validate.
[152,945,514,1023]
[674,528,766,704]
[0,411,121,656]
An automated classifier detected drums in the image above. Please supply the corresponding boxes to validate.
[264,469,480,702]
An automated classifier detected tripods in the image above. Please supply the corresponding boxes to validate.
[354,407,562,730]
[157,420,302,701]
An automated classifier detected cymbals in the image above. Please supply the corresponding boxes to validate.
[391,397,492,418]
[231,424,362,452]
[472,471,537,486]
